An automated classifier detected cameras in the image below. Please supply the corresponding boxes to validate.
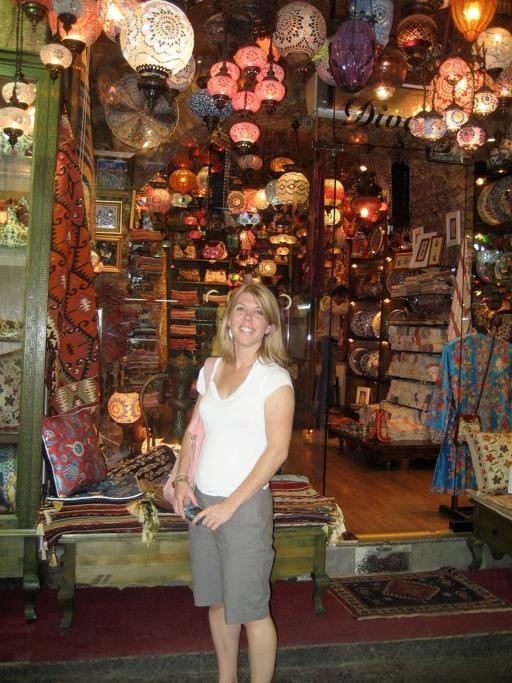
[184,506,206,527]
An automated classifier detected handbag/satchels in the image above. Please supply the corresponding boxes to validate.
[161,356,217,509]
[451,413,483,444]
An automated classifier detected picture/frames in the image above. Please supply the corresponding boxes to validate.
[445,210,461,248]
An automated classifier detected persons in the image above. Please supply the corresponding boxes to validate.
[165,281,295,682]
[308,285,349,415]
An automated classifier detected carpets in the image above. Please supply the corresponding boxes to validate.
[327,566,512,622]
[0,567,512,666]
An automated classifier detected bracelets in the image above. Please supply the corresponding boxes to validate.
[171,475,188,487]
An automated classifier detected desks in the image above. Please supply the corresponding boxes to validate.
[57,526,330,632]
[467,493,512,571]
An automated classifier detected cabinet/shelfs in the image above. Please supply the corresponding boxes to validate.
[0,51,64,623]
[120,203,455,470]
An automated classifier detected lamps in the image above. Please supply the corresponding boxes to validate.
[107,379,141,460]
[0,0,512,277]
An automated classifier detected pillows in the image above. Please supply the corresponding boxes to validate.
[42,409,108,498]
